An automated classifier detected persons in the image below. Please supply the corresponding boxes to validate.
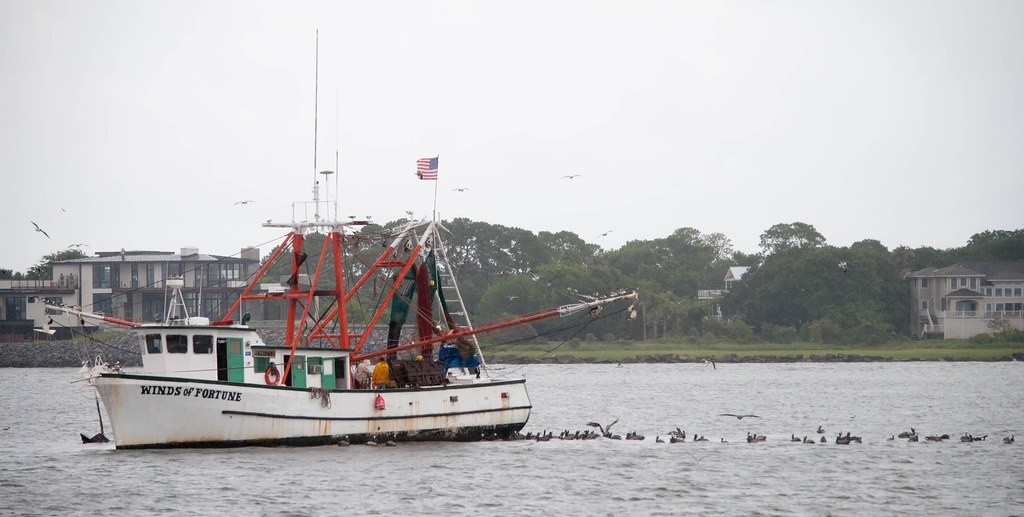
[354,359,373,390]
[371,356,390,390]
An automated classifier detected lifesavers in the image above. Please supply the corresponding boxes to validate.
[265,367,280,386]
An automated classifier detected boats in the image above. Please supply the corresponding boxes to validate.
[31,155,638,449]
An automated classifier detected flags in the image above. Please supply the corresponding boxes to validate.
[416,156,439,180]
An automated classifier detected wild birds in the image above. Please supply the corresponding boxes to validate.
[541,347,718,372]
[389,412,1015,447]
[562,174,614,240]
[413,170,470,193]
[529,269,553,287]
[837,258,850,275]
[504,295,520,302]
[29,207,91,250]
[231,198,254,206]
